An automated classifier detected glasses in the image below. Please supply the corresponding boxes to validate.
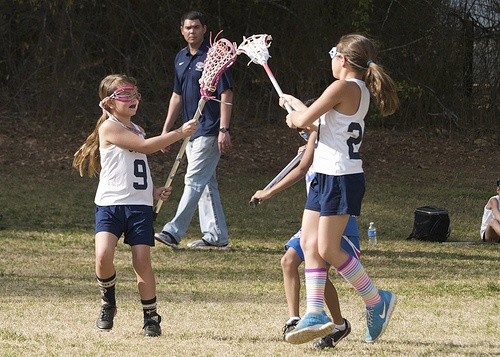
[109,85,142,104]
[328,46,344,60]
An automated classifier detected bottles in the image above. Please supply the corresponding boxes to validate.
[368,222,377,246]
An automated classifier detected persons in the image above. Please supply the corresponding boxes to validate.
[480,179,500,243]
[73,73,200,336]
[250,98,352,350]
[153,10,233,248]
[278,35,398,345]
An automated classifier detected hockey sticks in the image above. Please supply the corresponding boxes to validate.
[150,37,238,223]
[236,32,294,123]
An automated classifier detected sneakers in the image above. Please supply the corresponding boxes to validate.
[188,238,231,251]
[315,319,352,350]
[281,318,300,342]
[285,311,336,345]
[155,230,179,250]
[142,312,163,338]
[363,288,398,343]
[96,302,117,330]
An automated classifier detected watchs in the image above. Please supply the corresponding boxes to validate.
[219,127,230,133]
